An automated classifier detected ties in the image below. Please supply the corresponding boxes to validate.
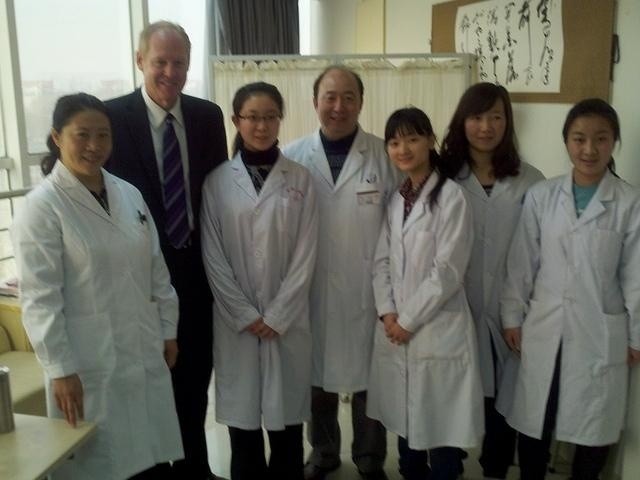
[162,115,189,251]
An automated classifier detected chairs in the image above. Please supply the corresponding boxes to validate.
[0,302,47,416]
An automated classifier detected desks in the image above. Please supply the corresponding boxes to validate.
[0,412,98,480]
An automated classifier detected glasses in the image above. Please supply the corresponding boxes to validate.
[237,114,284,123]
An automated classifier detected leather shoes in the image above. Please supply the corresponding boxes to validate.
[304,460,341,480]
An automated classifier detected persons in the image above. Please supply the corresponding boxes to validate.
[101,18,239,480]
[361,101,486,479]
[8,90,187,480]
[498,97,640,479]
[278,62,406,479]
[436,79,547,480]
[202,79,321,479]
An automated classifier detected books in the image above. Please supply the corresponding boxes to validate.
[1,271,21,298]
